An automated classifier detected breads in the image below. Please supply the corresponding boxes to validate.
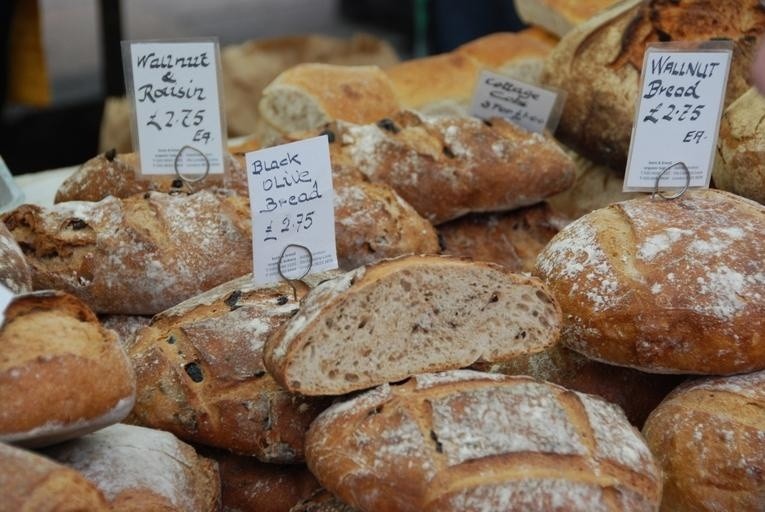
[0,0,765,512]
